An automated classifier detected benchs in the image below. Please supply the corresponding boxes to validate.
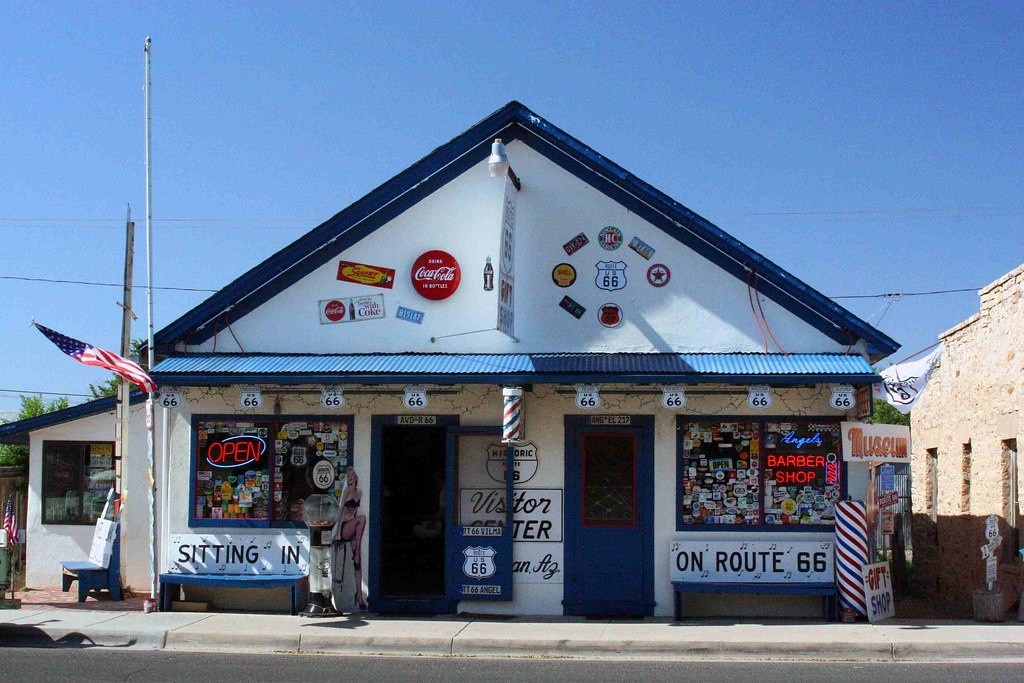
[156,536,311,616]
[60,517,122,602]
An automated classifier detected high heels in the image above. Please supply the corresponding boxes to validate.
[355,593,366,610]
[352,550,361,570]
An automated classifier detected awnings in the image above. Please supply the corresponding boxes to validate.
[532,353,884,386]
[146,352,536,386]
[0,419,29,445]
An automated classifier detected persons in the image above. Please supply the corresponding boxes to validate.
[333,467,367,609]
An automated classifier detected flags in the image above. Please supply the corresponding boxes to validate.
[3,494,21,546]
[33,321,157,393]
